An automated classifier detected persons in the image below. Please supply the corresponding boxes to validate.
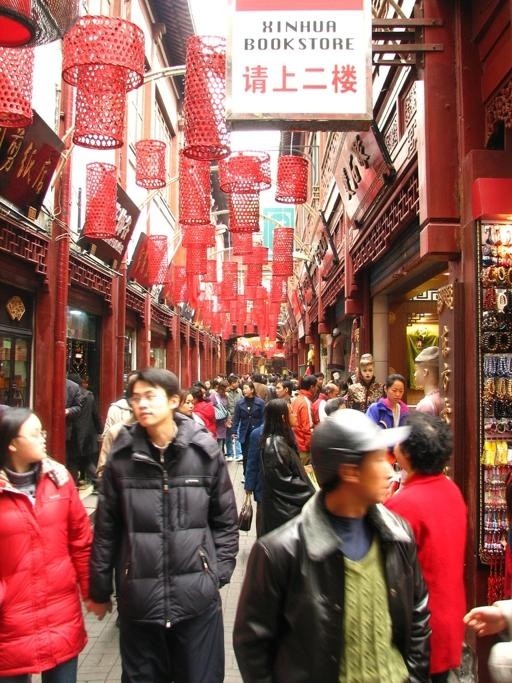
[217,381,233,463]
[0,408,94,682]
[267,371,291,378]
[102,370,139,437]
[275,379,293,405]
[384,412,467,682]
[290,375,317,467]
[365,374,410,429]
[175,390,206,427]
[231,382,266,483]
[224,376,244,462]
[232,407,431,683]
[315,372,324,388]
[242,373,252,382]
[260,399,316,537]
[325,397,346,416]
[347,353,383,410]
[85,368,238,683]
[192,375,222,388]
[244,423,262,540]
[413,345,441,412]
[311,383,339,425]
[268,378,275,395]
[461,600,511,683]
[95,413,133,477]
[252,374,266,398]
[65,374,99,488]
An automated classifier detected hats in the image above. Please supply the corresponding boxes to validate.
[308,408,413,489]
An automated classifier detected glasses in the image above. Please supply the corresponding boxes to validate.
[11,431,49,444]
[126,393,168,407]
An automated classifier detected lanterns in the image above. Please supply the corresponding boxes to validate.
[242,257,268,299]
[201,259,217,283]
[61,15,144,150]
[135,140,166,190]
[214,283,224,303]
[272,227,293,276]
[275,155,308,204]
[232,231,253,255]
[183,33,232,162]
[218,149,271,232]
[173,266,189,303]
[84,162,116,239]
[201,300,279,342]
[0,0,36,128]
[182,224,217,275]
[223,262,238,298]
[271,276,288,303]
[147,235,171,286]
[179,149,210,226]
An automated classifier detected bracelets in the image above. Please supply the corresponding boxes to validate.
[481,223,512,433]
[483,438,511,606]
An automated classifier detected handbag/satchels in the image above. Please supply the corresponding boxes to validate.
[237,493,254,531]
[213,394,229,422]
